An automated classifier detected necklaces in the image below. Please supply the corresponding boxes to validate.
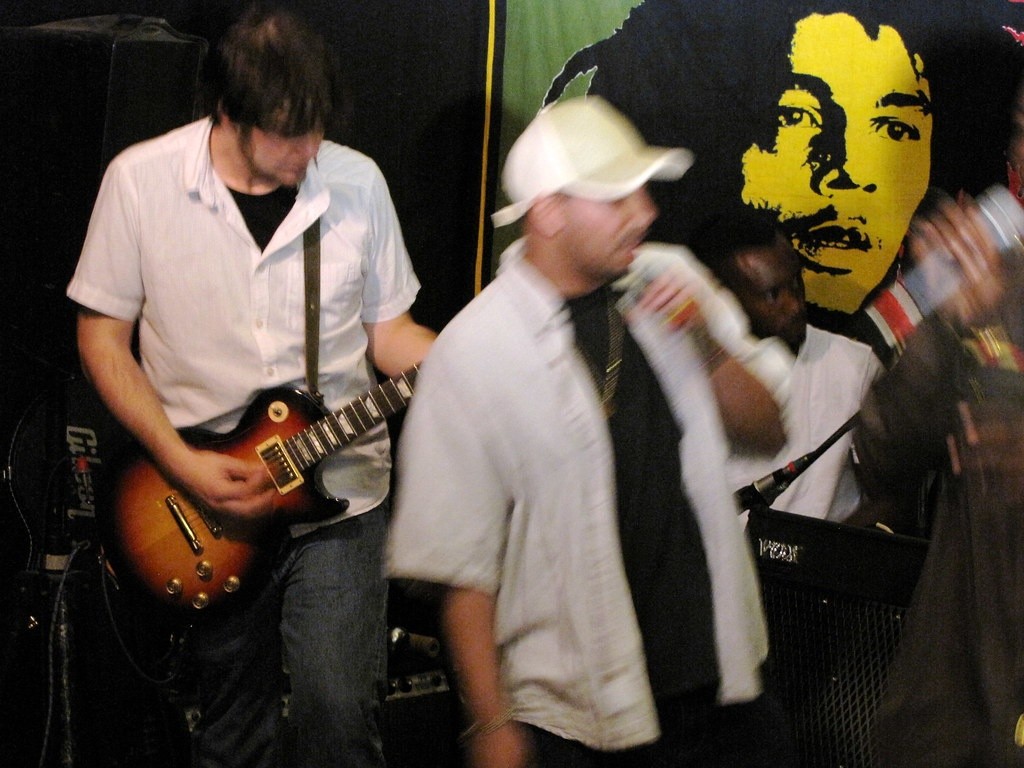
[569,291,621,423]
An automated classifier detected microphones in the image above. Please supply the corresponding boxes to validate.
[735,451,819,512]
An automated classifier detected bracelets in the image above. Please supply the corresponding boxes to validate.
[457,707,517,749]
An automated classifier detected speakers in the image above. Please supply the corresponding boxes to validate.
[184,669,464,768]
[745,507,934,768]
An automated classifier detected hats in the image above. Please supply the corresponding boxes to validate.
[491,95,694,228]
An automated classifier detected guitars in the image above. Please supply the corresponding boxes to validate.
[103,358,426,611]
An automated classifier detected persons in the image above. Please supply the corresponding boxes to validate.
[858,90,1023,768]
[386,94,790,768]
[709,227,882,531]
[74,9,442,768]
